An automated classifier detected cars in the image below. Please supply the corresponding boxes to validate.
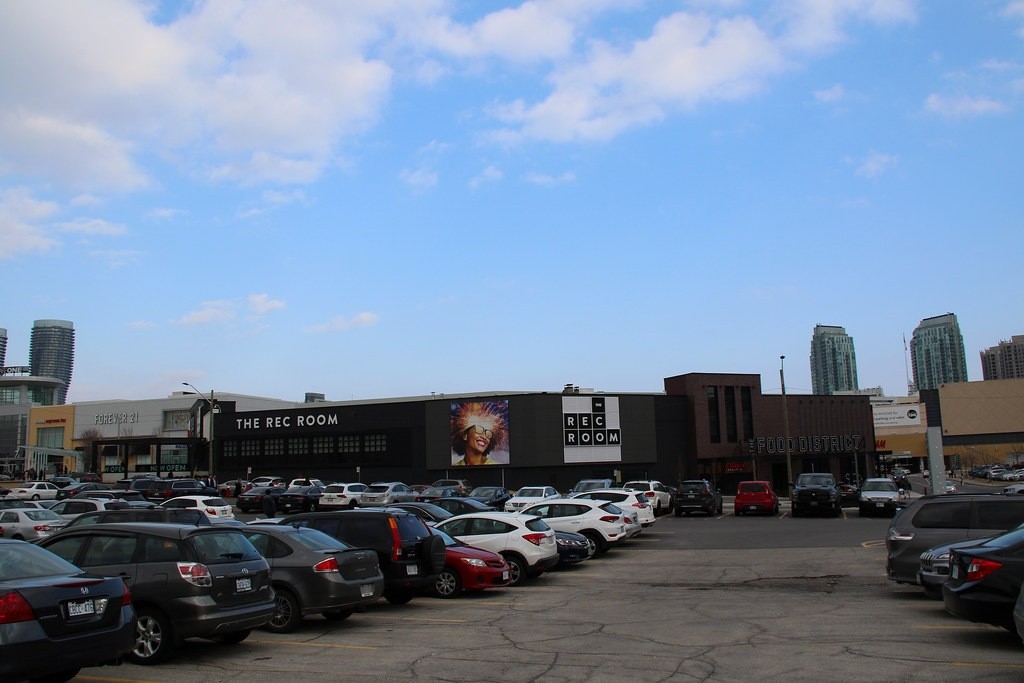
[922,469,931,478]
[946,480,957,493]
[734,481,779,516]
[971,463,1024,481]
[859,477,900,515]
[1001,483,1024,494]
[890,466,911,482]
[919,519,1024,645]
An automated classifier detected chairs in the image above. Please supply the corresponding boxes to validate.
[101,542,123,562]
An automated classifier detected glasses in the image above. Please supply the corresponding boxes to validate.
[464,425,494,439]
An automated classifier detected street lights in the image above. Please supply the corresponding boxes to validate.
[181,382,214,475]
[778,355,793,501]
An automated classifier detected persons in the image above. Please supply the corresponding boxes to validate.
[64,466,68,474]
[904,478,912,499]
[302,476,310,486]
[27,467,43,480]
[269,480,275,486]
[262,489,276,518]
[960,473,963,486]
[453,415,497,465]
[247,480,254,490]
[225,483,231,497]
[205,474,217,489]
[948,469,956,478]
[234,477,242,497]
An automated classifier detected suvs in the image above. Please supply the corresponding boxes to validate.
[788,473,842,517]
[673,479,723,517]
[885,493,1024,586]
[0,471,671,683]
